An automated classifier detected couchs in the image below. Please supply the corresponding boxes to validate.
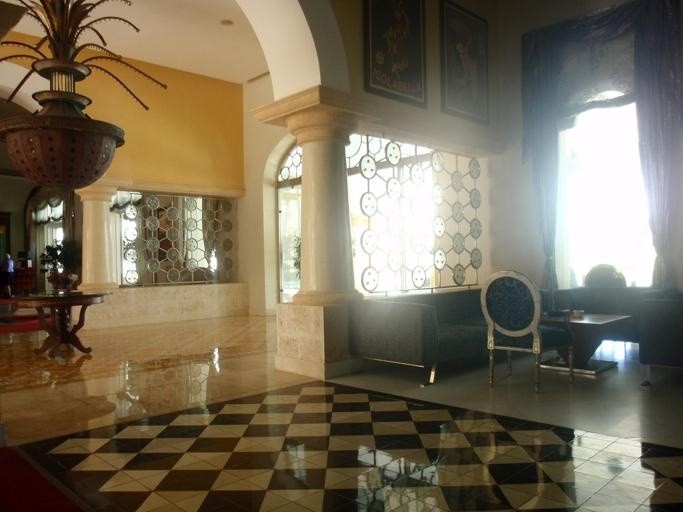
[356,288,549,387]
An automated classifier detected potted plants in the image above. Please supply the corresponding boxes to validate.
[0,1,166,189]
[40,237,82,292]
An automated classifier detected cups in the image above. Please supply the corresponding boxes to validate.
[573,310,584,318]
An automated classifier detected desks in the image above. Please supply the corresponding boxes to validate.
[16,291,111,354]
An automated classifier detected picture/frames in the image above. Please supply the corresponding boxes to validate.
[359,0,489,122]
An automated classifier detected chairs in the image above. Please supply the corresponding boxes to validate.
[480,271,574,391]
[638,290,683,388]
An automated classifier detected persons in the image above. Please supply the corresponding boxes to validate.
[2,253,14,299]
